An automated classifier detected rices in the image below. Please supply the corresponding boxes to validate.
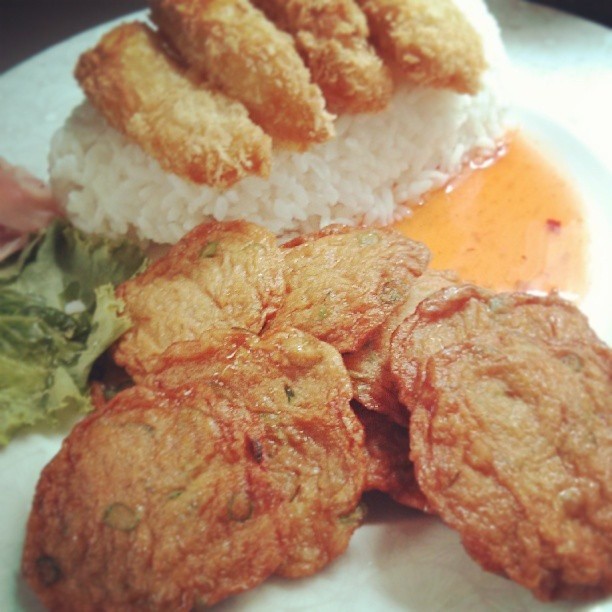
[48,0,521,245]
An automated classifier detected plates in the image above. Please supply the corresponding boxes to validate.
[1,1,611,611]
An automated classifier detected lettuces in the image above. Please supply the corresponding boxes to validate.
[0,153,140,445]
